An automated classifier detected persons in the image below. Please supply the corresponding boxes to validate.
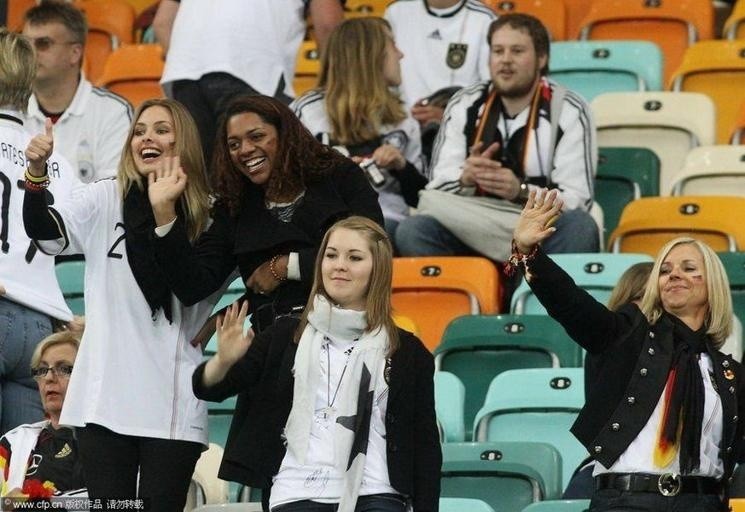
[18,1,134,185]
[286,16,427,258]
[22,100,253,512]
[192,216,443,512]
[0,315,89,512]
[560,259,657,500]
[151,0,346,172]
[148,96,385,333]
[0,25,85,437]
[503,188,745,511]
[383,1,499,162]
[393,12,597,258]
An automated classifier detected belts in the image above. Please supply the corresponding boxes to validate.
[595,473,723,493]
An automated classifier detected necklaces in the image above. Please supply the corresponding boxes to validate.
[323,301,360,420]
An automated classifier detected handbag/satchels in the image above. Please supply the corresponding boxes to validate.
[417,190,543,264]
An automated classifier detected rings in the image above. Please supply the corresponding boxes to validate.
[155,177,163,182]
[258,289,266,295]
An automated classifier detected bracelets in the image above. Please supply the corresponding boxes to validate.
[23,167,50,191]
[512,239,540,261]
[270,254,288,282]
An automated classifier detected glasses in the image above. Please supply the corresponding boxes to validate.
[33,38,81,50]
[31,366,73,375]
